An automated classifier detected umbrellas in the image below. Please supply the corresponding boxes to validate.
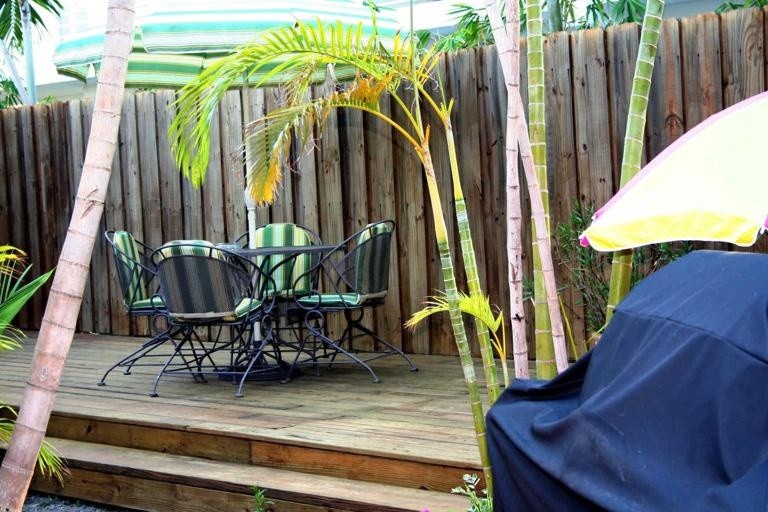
[579,89,764,253]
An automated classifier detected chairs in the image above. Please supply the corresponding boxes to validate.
[281,220,421,383]
[230,222,326,382]
[98,230,219,386]
[149,238,284,398]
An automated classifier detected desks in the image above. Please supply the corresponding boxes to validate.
[188,243,346,385]
